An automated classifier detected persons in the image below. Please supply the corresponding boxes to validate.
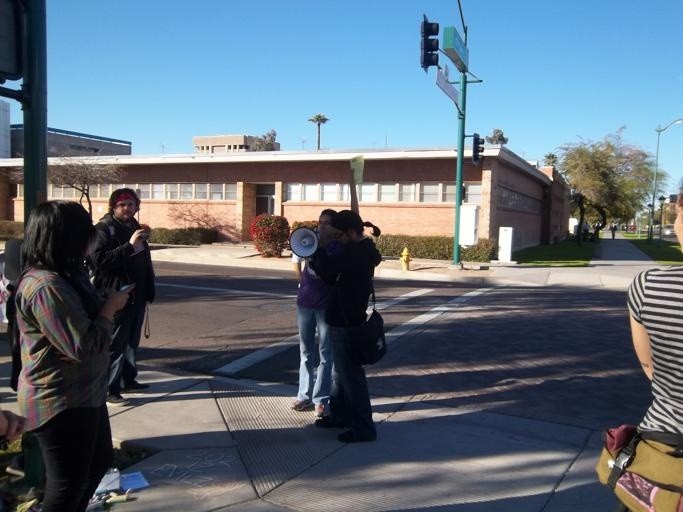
[0,410,27,447]
[313,209,381,443]
[626,184,682,512]
[582,223,590,236]
[85,188,155,407]
[608,221,618,240]
[291,209,335,420]
[13,200,139,512]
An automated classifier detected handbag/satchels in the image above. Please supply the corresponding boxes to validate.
[595,424,683,512]
[350,310,387,366]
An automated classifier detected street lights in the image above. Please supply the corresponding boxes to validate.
[647,118,683,241]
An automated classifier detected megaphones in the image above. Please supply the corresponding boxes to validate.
[288,227,322,258]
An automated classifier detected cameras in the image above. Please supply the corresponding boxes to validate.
[139,232,149,241]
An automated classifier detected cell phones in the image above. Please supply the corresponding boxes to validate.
[118,284,131,292]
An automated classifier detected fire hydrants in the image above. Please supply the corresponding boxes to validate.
[400,246,411,269]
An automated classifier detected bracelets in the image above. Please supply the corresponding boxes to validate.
[2,411,11,438]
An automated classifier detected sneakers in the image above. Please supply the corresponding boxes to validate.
[314,403,327,419]
[316,416,344,428]
[123,382,151,393]
[290,398,313,411]
[105,395,131,407]
[338,430,377,443]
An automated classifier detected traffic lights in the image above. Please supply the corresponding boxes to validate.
[473,132,485,162]
[419,12,440,75]
